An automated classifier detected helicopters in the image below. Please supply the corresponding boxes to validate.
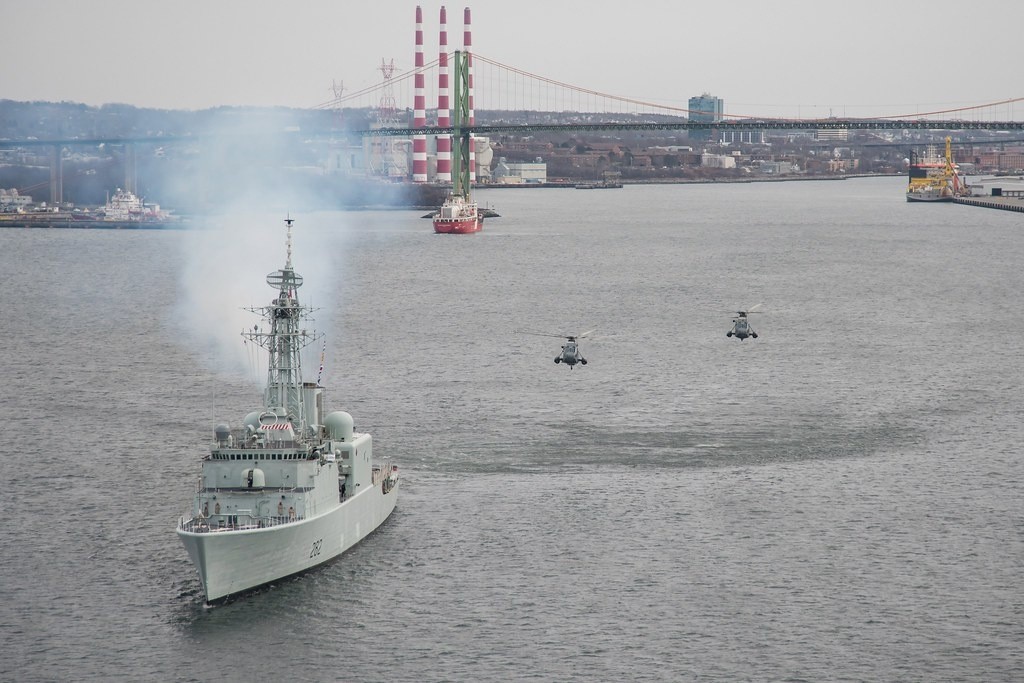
[516,326,610,370]
[704,302,774,341]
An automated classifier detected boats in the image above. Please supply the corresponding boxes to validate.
[904,142,959,185]
[598,170,624,189]
[175,215,403,608]
[576,184,594,189]
[432,192,484,234]
[906,187,953,202]
[71,189,171,222]
[424,203,501,218]
[908,134,954,189]
